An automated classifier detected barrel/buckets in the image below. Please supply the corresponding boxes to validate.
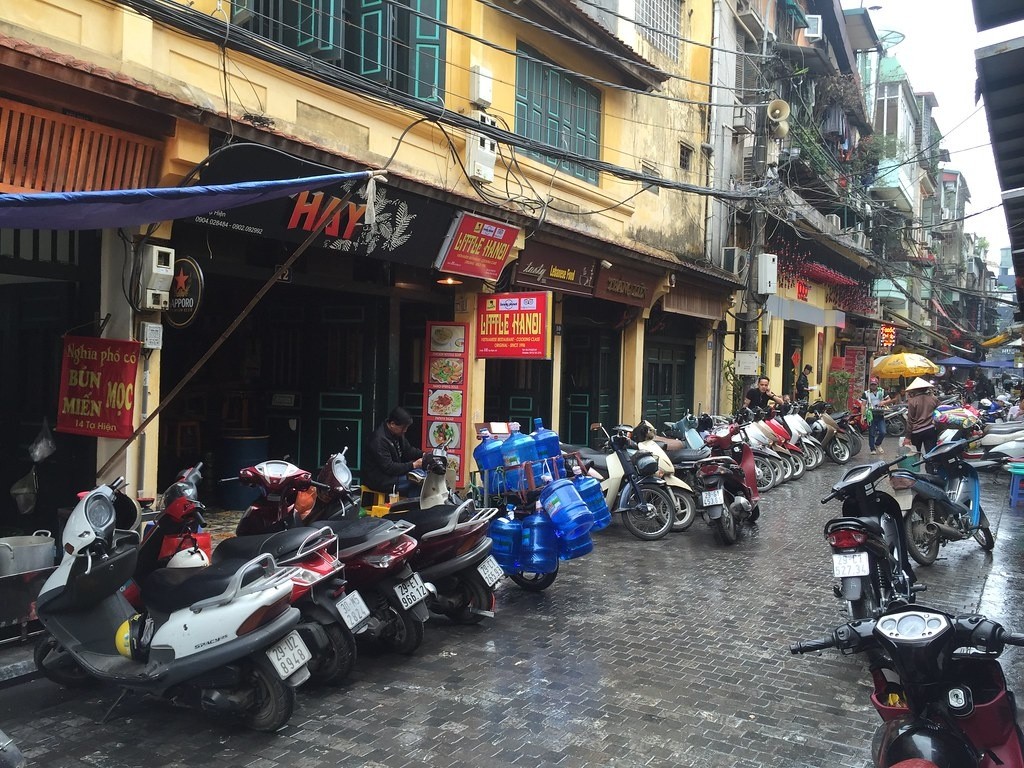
[214,433,270,512]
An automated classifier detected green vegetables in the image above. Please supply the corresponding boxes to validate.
[433,423,453,439]
[436,361,454,381]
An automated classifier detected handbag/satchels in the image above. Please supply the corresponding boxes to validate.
[158,526,211,560]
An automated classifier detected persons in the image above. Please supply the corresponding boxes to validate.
[986,394,1010,423]
[965,373,995,401]
[995,371,1009,388]
[902,377,939,454]
[742,375,783,410]
[360,407,426,498]
[796,364,815,402]
[860,377,891,454]
[1008,396,1024,421]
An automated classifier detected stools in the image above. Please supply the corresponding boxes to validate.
[1004,456,1024,507]
[360,485,386,516]
[162,419,202,457]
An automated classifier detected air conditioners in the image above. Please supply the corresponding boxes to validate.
[924,234,933,248]
[941,208,951,221]
[826,214,840,231]
[733,108,753,135]
[855,232,867,249]
[920,230,928,245]
[721,247,751,281]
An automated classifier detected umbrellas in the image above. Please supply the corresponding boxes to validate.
[870,352,939,401]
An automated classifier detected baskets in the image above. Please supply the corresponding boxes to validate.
[631,453,659,475]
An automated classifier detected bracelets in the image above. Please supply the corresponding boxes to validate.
[771,394,775,398]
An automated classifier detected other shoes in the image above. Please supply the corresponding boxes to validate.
[870,450,877,454]
[875,444,884,454]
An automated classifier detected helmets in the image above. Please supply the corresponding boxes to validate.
[113,492,142,531]
[168,547,210,568]
[637,455,657,474]
[634,420,656,442]
[812,419,826,433]
[114,613,148,664]
[885,722,981,768]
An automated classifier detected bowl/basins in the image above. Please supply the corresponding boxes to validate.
[432,327,453,345]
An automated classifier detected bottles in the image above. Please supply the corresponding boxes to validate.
[472,418,611,574]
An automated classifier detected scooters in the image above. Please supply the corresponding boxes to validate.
[601,383,909,532]
[936,373,1023,481]
[213,459,439,660]
[888,430,994,567]
[820,454,927,620]
[691,422,762,545]
[789,599,1024,768]
[552,422,676,542]
[314,445,519,628]
[118,460,370,691]
[409,437,574,593]
[31,475,314,735]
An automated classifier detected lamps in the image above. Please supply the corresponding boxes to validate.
[435,273,464,285]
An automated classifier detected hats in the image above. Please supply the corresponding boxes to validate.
[804,364,814,374]
[996,395,1011,406]
[905,376,936,393]
[869,376,879,385]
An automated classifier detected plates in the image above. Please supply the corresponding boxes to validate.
[429,422,460,448]
[428,388,462,417]
[431,360,462,384]
[447,453,459,481]
